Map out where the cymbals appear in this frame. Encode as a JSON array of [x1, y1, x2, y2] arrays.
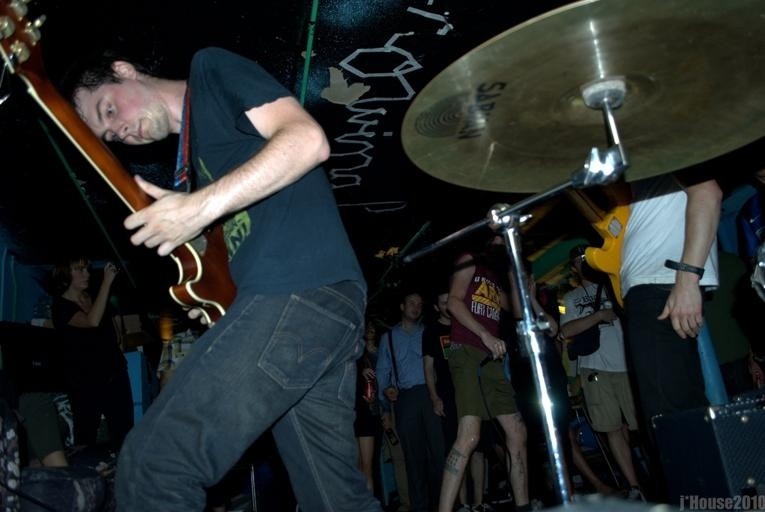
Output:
[[402, 0, 765, 195]]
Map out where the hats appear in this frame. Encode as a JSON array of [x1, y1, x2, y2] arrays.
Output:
[[570, 244, 589, 260]]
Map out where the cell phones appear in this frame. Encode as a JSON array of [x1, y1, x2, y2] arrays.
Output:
[[385, 428, 399, 446]]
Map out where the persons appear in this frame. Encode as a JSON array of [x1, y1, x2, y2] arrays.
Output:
[[52, 238, 133, 474], [62, 41, 387, 511], [156, 325, 201, 393], [17, 387, 79, 472]]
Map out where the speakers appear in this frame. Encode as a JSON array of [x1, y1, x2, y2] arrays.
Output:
[[0, 320, 70, 409], [650, 397, 765, 512]]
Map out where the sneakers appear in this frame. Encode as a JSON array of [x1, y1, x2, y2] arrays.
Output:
[[455, 502, 494, 511], [626, 486, 644, 502]]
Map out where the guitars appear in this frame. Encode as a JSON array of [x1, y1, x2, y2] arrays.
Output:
[[565, 187, 631, 307], [0, 0, 236, 328]]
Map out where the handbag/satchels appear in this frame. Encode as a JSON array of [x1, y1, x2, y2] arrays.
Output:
[[567, 321, 600, 361]]
